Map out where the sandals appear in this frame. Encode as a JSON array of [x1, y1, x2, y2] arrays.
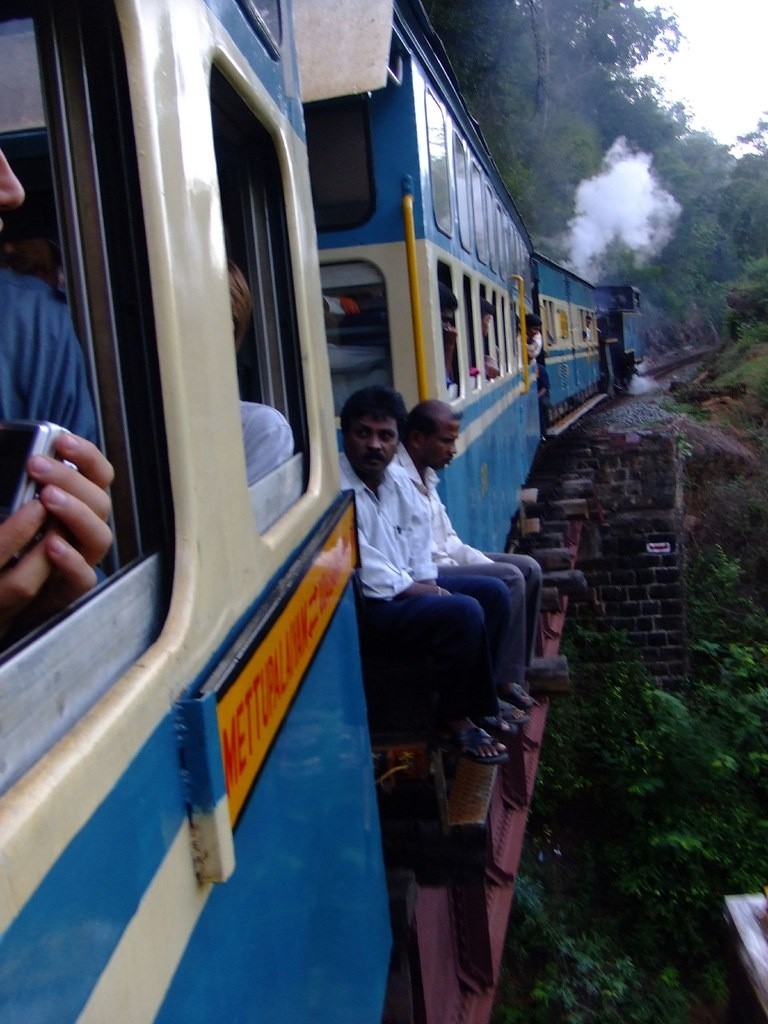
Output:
[[497, 698, 528, 724], [504, 683, 534, 709]]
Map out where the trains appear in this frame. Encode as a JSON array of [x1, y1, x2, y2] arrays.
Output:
[[1, 0, 644, 1024]]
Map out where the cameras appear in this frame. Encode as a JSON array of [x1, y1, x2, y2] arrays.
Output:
[[0, 419, 80, 562]]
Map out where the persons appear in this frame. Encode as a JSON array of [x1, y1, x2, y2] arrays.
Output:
[[0, 152, 593, 640], [395, 401, 542, 725], [336, 385, 522, 766]]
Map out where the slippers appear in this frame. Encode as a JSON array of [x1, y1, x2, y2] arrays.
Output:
[[471, 714, 516, 738], [430, 717, 510, 765]]
